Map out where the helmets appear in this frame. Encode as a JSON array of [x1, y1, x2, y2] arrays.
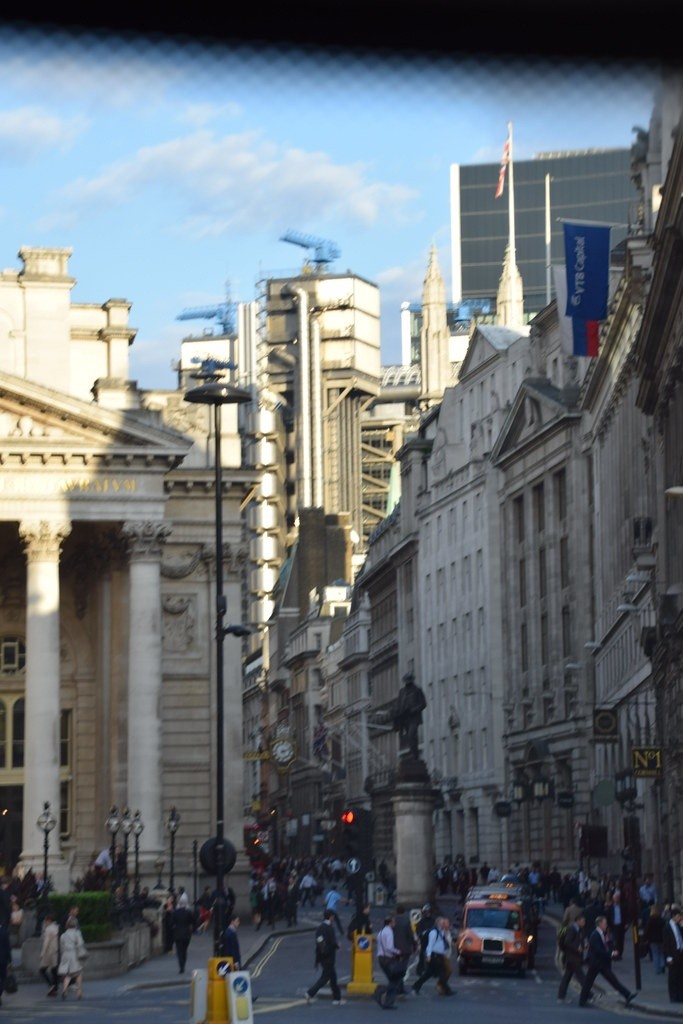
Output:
[[421, 903, 432, 915]]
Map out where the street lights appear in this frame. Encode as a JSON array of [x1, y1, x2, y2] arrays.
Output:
[[106, 805, 120, 897], [129, 810, 145, 896], [34, 801, 57, 935], [169, 805, 182, 898], [187, 379, 252, 884], [119, 809, 132, 899]]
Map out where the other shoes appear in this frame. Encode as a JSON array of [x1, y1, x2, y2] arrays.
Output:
[[588, 992, 603, 1002], [47, 983, 86, 1002], [332, 999, 348, 1007], [578, 1000, 596, 1009], [381, 1003, 397, 1010], [305, 992, 313, 1006], [625, 991, 640, 1004], [554, 996, 574, 1003], [410, 985, 423, 995], [442, 989, 458, 997], [178, 966, 185, 973], [373, 987, 382, 1006]]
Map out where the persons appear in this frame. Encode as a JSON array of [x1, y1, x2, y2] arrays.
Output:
[[37, 912, 58, 995], [57, 916, 86, 1000], [395, 903, 453, 994], [303, 909, 347, 1006], [58, 904, 80, 933], [434, 856, 682, 974], [555, 914, 599, 1003], [395, 672, 426, 756], [222, 914, 257, 1002], [0, 844, 149, 933], [578, 915, 638, 1007], [376, 915, 402, 1008], [163, 852, 397, 974], [37, 906, 50, 945], [663, 908, 682, 1003], [0, 919, 12, 1006]]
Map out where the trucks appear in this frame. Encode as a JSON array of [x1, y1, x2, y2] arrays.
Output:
[[458, 882, 544, 976]]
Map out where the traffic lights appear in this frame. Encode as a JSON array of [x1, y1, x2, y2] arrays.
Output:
[[337, 805, 371, 831]]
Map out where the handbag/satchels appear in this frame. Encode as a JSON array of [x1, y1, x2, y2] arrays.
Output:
[[385, 952, 402, 980], [74, 929, 90, 961], [5, 964, 19, 993]]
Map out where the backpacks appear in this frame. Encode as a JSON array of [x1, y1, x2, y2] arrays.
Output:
[[420, 926, 439, 951], [314, 924, 332, 958], [555, 926, 568, 951]]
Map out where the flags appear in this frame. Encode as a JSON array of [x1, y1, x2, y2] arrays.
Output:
[[562, 223, 609, 319], [495, 141, 508, 197], [572, 318, 598, 356], [313, 725, 328, 754]]
